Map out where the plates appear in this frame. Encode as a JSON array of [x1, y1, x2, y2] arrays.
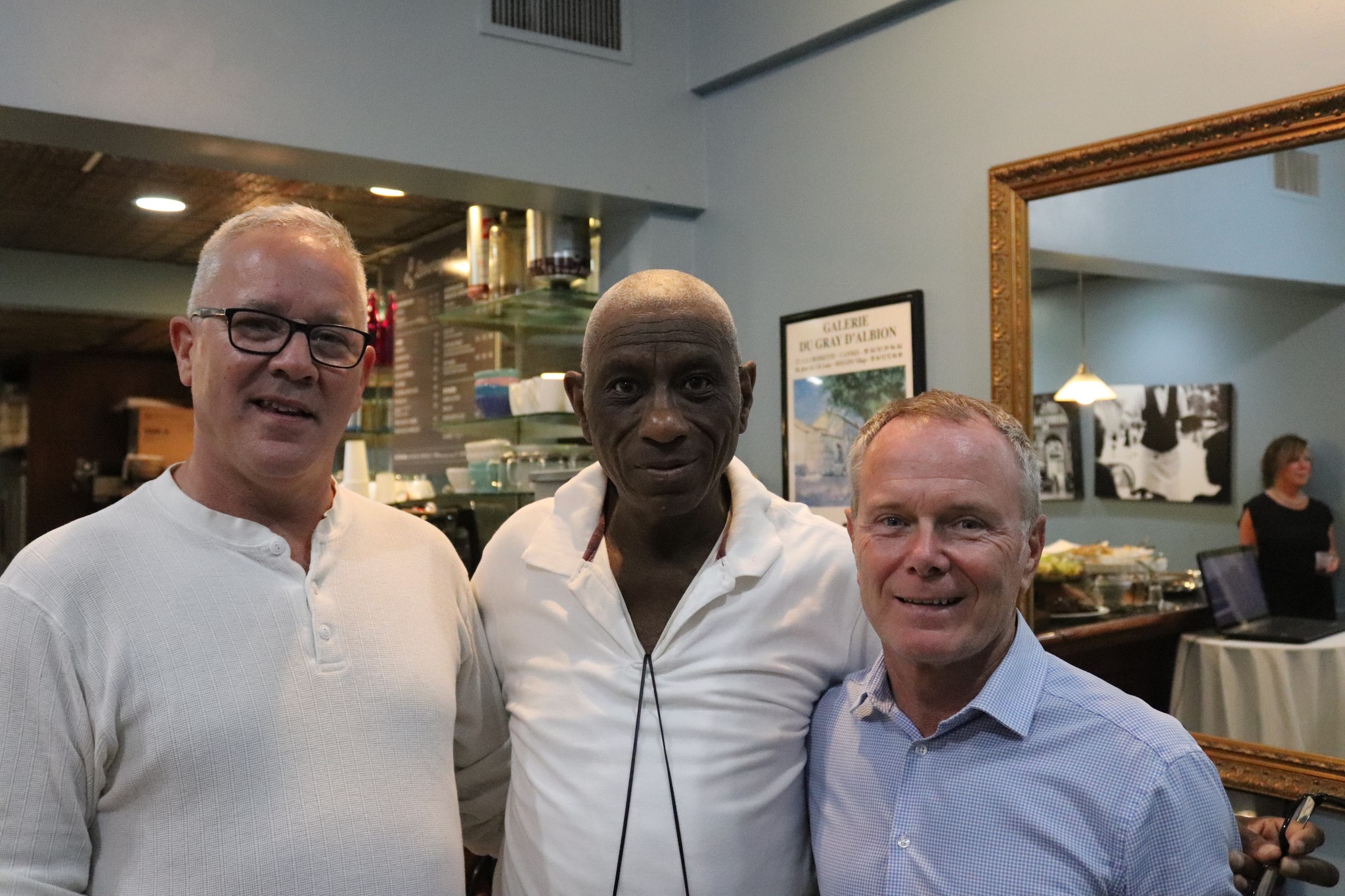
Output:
[[1157, 571, 1203, 595], [1050, 605, 1111, 618]]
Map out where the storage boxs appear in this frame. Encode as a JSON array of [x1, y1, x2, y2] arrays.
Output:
[[120, 407, 194, 462]]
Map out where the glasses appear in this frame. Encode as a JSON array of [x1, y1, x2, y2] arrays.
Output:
[[1249, 788, 1345, 896], [189, 305, 375, 369]]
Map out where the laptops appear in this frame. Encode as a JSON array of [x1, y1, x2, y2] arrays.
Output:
[[1196, 546, 1345, 645]]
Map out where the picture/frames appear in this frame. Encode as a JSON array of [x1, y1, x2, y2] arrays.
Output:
[[778, 289, 928, 525]]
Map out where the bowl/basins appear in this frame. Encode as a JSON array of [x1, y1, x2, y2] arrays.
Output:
[[473, 369, 518, 417], [445, 466, 473, 494]]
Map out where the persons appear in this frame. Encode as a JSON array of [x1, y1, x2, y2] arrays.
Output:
[[811, 388, 1243, 896], [467, 268, 1340, 896], [1236, 433, 1340, 621], [0, 204, 513, 896]]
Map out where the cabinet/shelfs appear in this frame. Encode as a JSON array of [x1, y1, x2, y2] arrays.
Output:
[[433, 287, 600, 439]]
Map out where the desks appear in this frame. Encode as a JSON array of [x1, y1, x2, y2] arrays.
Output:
[[1169, 634, 1345, 761], [1033, 607, 1216, 715]]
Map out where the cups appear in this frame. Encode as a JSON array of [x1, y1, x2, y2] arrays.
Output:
[[342, 439, 395, 505], [508, 378, 574, 415], [467, 456, 592, 493]]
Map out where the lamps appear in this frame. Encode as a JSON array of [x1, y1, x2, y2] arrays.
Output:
[[1053, 272, 1118, 407]]
